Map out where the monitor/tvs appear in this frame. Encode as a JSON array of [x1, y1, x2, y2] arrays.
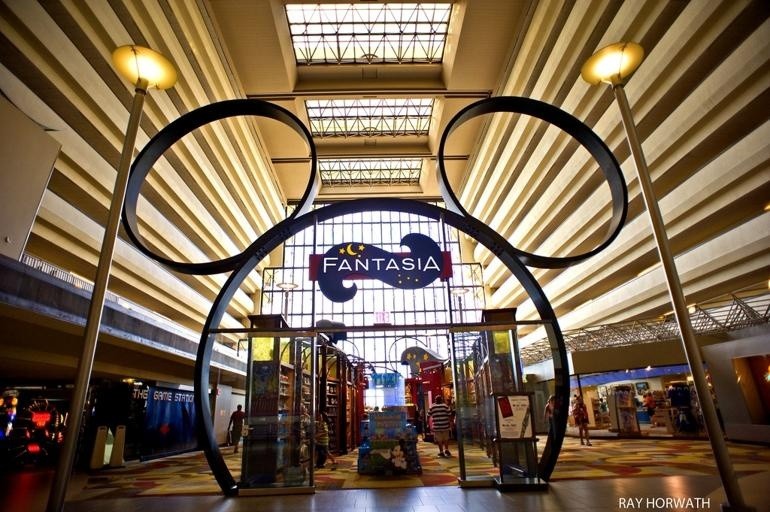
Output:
[[636, 383, 648, 390]]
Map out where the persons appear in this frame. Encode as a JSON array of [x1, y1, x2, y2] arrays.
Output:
[[598, 398, 608, 415], [311, 411, 338, 471], [543, 395, 556, 424], [227, 404, 246, 454], [642, 391, 658, 428], [572, 395, 593, 446], [425, 395, 453, 457]]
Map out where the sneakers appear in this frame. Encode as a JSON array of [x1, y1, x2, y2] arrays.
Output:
[[580, 441, 592, 446], [437, 450, 452, 457]]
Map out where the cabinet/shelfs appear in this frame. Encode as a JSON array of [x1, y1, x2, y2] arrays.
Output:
[[240, 359, 359, 485], [474, 353, 537, 476]]
